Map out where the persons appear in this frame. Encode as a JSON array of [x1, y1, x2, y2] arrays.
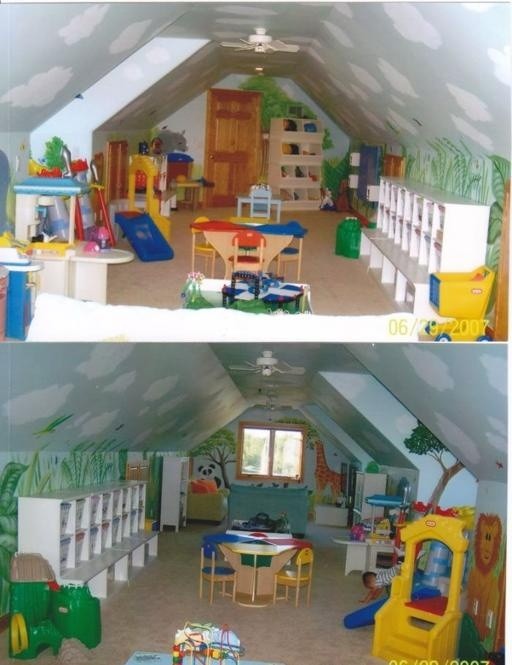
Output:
[[357, 564, 400, 604]]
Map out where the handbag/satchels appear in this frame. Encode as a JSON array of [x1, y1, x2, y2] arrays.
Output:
[[245, 513, 277, 532]]
